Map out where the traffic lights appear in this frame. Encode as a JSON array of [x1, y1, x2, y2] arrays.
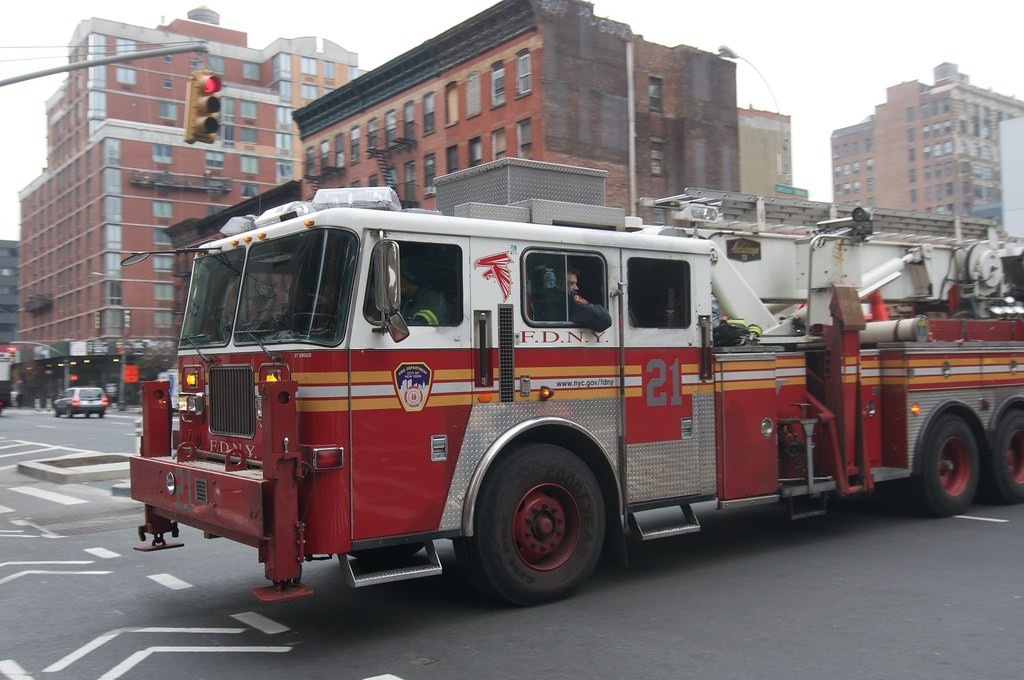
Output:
[[183, 70, 223, 144]]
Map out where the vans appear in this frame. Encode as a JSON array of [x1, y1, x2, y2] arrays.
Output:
[[53, 388, 108, 418]]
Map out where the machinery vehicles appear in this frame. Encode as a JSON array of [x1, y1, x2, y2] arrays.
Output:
[[128, 183, 1024, 605]]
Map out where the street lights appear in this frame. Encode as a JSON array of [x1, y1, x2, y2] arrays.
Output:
[[718, 45, 792, 190], [92, 272, 127, 411]]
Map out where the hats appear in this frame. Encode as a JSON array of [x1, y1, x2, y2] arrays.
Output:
[[404, 259, 432, 289]]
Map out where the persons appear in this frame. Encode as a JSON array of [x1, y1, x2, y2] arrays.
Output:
[[308, 255, 456, 325], [535, 266, 612, 332]]
